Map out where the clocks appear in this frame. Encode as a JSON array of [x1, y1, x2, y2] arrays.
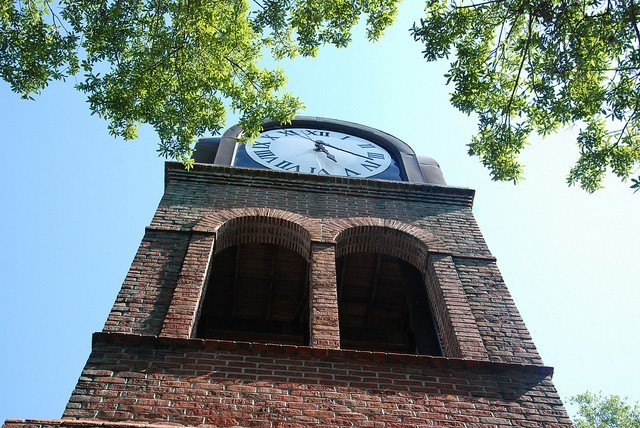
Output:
[[230, 120, 409, 182]]
